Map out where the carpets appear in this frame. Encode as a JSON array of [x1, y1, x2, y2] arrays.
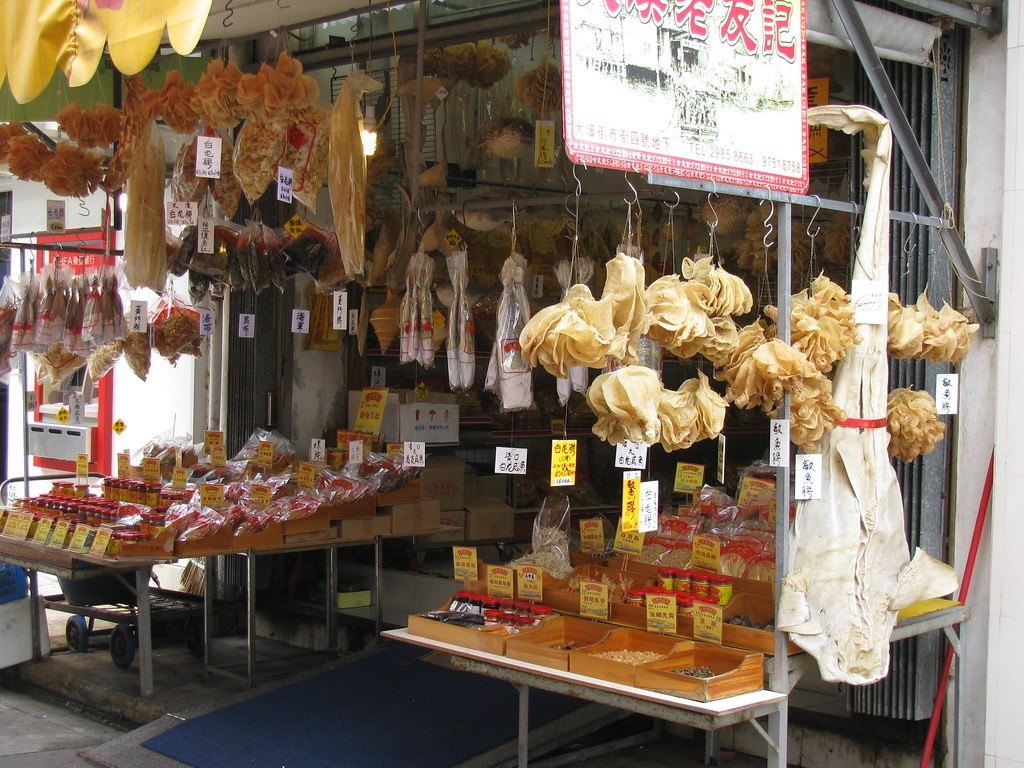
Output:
[[141, 637, 590, 768]]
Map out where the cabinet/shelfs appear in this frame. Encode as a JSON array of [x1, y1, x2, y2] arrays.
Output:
[[352, 278, 772, 521]]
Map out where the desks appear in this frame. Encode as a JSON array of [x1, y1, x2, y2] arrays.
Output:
[[0, 535, 385, 695], [447, 653, 811, 767]]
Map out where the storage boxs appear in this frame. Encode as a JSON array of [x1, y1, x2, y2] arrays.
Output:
[[209, 386, 527, 542]]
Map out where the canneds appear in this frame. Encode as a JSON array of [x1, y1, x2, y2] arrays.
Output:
[[452, 591, 551, 634], [624, 567, 733, 617], [0, 476, 187, 555]]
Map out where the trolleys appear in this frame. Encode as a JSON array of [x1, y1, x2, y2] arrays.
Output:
[[0, 472, 238, 667]]
